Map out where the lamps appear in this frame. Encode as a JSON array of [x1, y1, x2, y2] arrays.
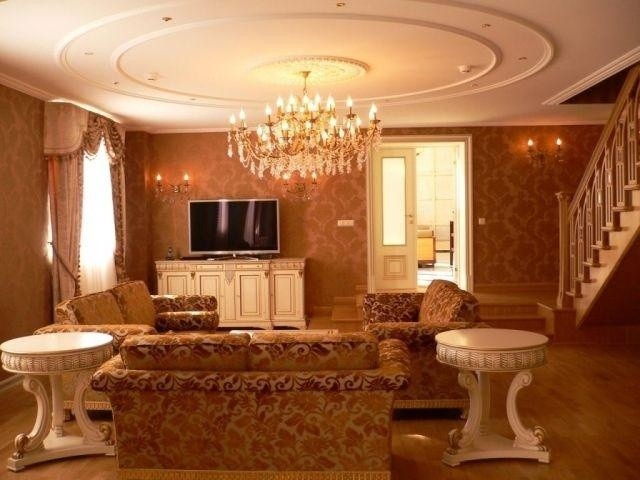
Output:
[[228, 53, 382, 176], [281, 172, 321, 205], [154, 172, 193, 206]]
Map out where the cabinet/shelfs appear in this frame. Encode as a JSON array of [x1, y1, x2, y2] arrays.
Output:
[[225, 259, 273, 331], [155, 258, 224, 330], [267, 258, 309, 331]]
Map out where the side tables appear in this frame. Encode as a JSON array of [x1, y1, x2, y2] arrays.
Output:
[[0, 331, 115, 472], [435, 328, 551, 467]]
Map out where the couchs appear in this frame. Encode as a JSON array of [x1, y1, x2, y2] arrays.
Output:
[[363, 280, 494, 420], [34, 280, 219, 421], [90, 331, 411, 479], [526, 137, 565, 177]]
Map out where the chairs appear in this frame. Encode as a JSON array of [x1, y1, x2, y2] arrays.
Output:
[[415, 231, 436, 267]]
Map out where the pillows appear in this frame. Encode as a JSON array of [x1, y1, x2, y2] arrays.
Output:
[[156, 311, 218, 330]]
